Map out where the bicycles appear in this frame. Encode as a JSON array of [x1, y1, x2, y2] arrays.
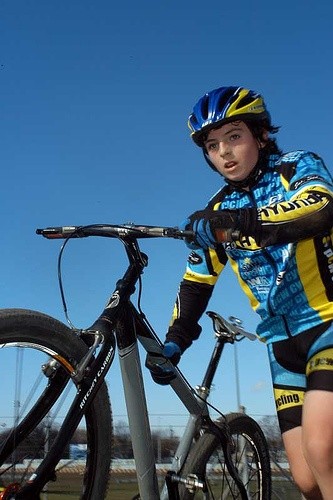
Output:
[[0, 222, 273, 500]]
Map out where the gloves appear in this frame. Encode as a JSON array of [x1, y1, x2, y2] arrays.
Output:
[[145, 331, 192, 386], [184, 207, 258, 250]]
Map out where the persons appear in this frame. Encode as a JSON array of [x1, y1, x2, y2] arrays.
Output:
[[145, 86, 333, 500]]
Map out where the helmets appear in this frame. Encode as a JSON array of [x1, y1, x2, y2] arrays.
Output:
[[188, 86, 266, 147]]
